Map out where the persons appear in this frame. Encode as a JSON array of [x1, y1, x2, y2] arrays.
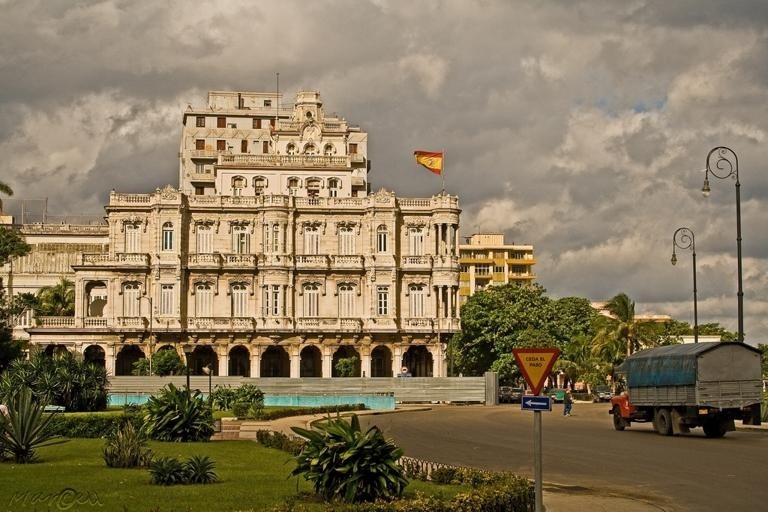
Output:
[[562, 388, 572, 417]]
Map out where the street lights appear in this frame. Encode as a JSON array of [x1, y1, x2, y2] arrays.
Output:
[[201, 363, 213, 422], [701, 145, 744, 345], [670, 227, 698, 344]]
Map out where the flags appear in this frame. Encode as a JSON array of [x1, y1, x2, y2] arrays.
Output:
[[413, 149, 443, 176]]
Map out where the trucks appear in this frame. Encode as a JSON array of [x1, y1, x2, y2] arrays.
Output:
[[607, 342, 763, 438]]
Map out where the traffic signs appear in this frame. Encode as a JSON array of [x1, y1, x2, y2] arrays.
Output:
[[511, 346, 560, 395], [520, 396, 550, 412]]
